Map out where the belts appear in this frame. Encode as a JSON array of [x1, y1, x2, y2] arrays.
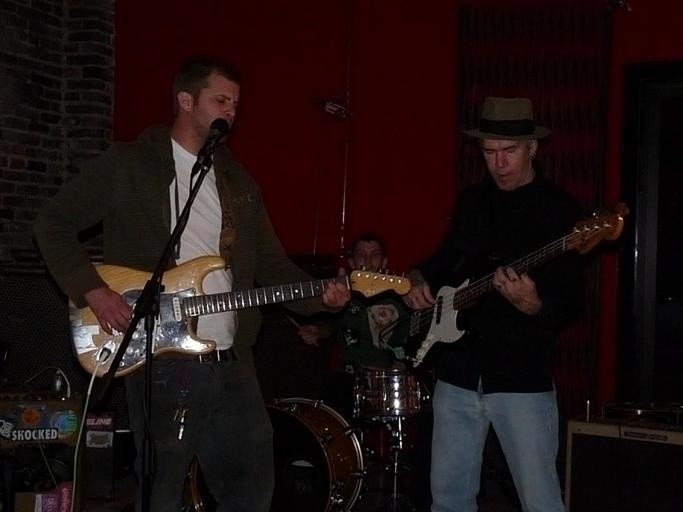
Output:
[[197, 346, 237, 364]]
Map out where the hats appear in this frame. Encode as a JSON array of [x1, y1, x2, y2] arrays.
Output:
[[457, 95, 554, 141]]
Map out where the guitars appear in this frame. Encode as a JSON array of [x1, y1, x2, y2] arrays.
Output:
[[378, 203, 630, 366], [70, 256, 411, 378]]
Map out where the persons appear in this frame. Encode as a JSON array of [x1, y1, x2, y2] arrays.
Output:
[[296, 231, 413, 428], [397, 97, 598, 512], [31, 53, 353, 512]]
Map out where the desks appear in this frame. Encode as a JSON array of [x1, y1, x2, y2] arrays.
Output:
[[564, 419, 682, 512]]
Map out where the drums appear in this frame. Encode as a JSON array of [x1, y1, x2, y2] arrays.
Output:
[[356, 368, 424, 419], [188, 397, 366, 509]]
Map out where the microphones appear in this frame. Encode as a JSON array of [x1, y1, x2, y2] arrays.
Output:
[[191, 118, 229, 176]]
